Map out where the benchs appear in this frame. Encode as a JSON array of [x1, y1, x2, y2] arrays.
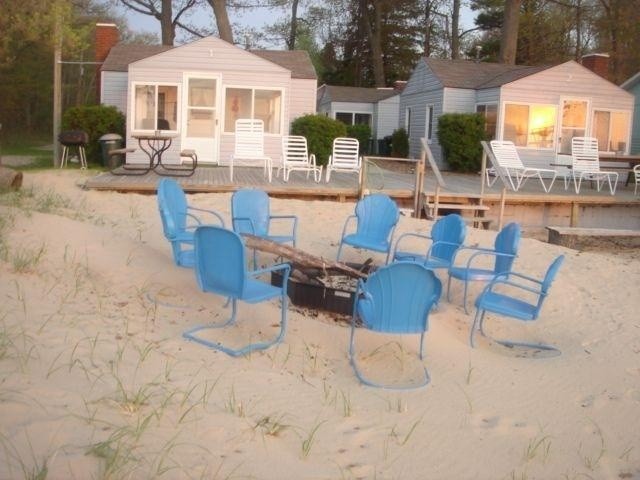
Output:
[[550, 163, 640, 174], [106, 147, 139, 176], [180, 148, 198, 178]]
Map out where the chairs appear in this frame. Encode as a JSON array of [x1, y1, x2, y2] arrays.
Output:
[[182, 225, 290, 358], [349, 262, 442, 390], [471, 256, 565, 359], [633, 164, 640, 194], [481, 139, 558, 192], [278, 136, 324, 183], [564, 137, 619, 196], [322, 136, 363, 182], [335, 192, 520, 315], [228, 118, 273, 182], [157, 178, 298, 269]]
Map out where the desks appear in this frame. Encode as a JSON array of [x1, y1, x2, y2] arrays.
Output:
[[131, 131, 180, 173], [558, 151, 640, 189]]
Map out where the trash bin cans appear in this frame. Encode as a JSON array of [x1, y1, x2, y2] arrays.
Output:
[[368, 136, 393, 157], [99, 133, 123, 168]]
